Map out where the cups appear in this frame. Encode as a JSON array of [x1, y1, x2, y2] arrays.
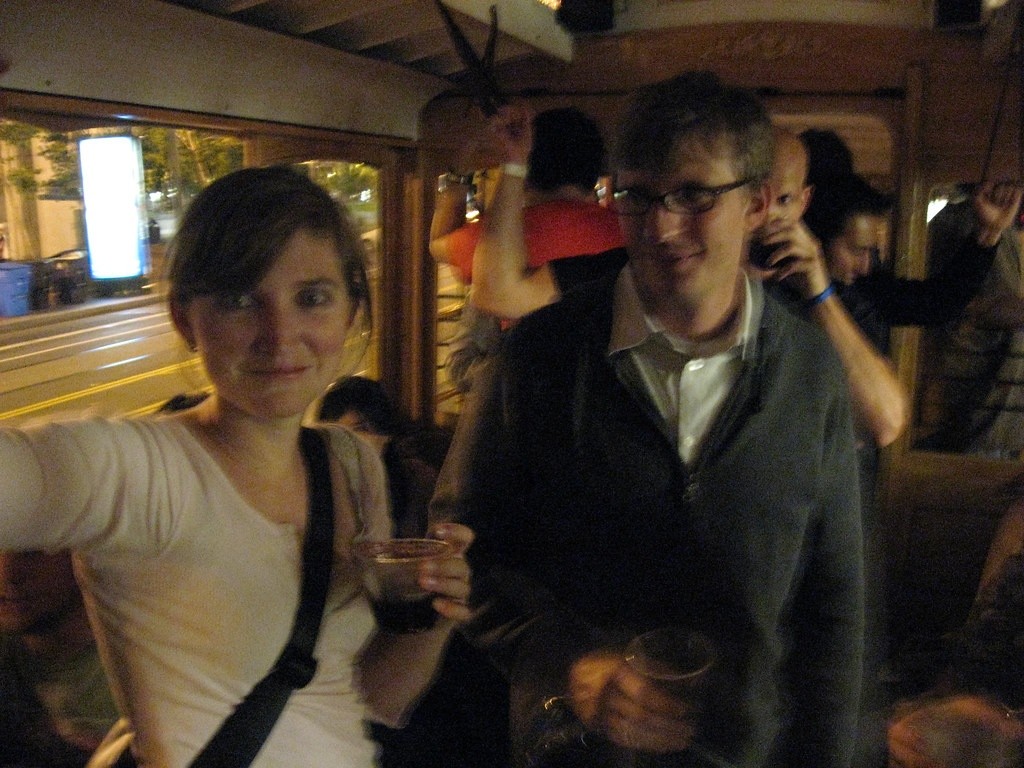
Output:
[[622, 627, 719, 714], [350, 538, 454, 636], [748, 232, 797, 271], [890, 692, 1022, 768]]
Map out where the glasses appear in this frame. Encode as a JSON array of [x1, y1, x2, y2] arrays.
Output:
[[607, 177, 758, 218]]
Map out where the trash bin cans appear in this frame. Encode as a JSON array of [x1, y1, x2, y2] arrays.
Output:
[[0, 262, 33, 317]]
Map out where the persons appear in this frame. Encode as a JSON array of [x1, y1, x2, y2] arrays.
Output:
[[0, 71, 1023, 768]]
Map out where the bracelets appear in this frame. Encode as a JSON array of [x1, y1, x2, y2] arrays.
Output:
[[439, 171, 471, 186], [801, 276, 838, 307], [502, 162, 529, 177]]
